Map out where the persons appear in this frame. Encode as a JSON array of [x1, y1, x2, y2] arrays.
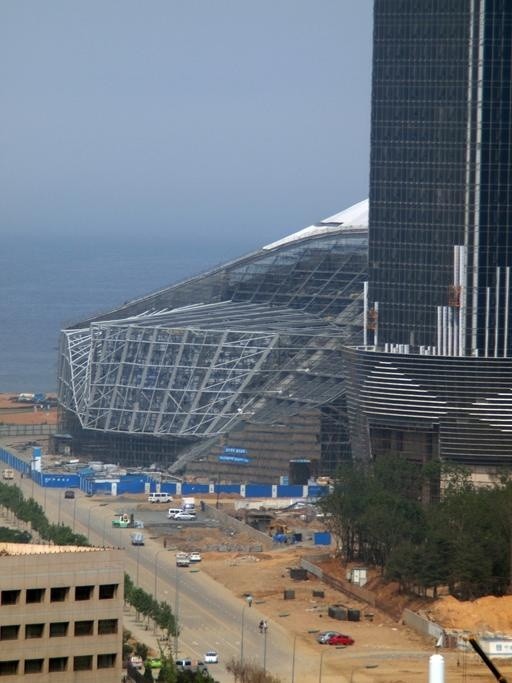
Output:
[[258, 620, 263, 633], [247, 594, 252, 608], [263, 620, 268, 633]]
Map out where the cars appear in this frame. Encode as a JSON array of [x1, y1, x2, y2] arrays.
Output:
[[316, 630, 340, 644], [144, 655, 164, 669], [190, 551, 202, 561], [39, 396, 58, 407], [174, 511, 197, 520], [202, 650, 221, 664], [329, 633, 356, 646]]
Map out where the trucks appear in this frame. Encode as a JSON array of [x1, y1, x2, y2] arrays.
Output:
[[174, 551, 192, 567], [128, 532, 145, 544], [181, 497, 196, 514]]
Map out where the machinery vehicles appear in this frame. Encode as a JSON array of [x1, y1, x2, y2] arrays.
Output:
[[460, 631, 509, 682], [16, 390, 46, 403], [268, 500, 327, 544], [112, 511, 136, 528]]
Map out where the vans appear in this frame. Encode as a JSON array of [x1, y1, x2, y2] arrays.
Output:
[[168, 507, 184, 519], [126, 653, 143, 670], [148, 492, 174, 503], [175, 658, 210, 676], [2, 468, 14, 479]]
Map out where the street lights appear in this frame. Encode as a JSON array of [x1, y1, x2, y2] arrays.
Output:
[[101, 511, 124, 547], [240, 600, 266, 669], [262, 611, 290, 676], [135, 535, 160, 617], [292, 626, 321, 682], [173, 567, 200, 660], [318, 644, 348, 682], [350, 663, 378, 682], [18, 457, 110, 545], [117, 522, 140, 551], [152, 545, 177, 631]]
